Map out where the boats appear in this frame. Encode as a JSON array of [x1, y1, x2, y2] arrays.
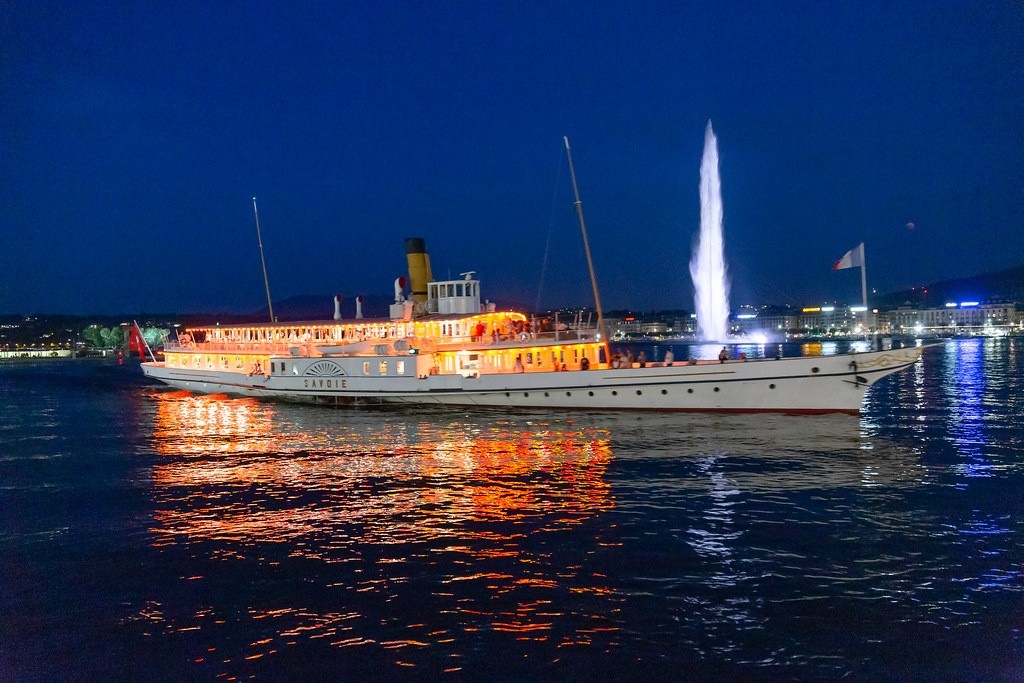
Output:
[[140, 136, 922, 412]]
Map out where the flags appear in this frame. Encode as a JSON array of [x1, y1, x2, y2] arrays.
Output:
[[128, 324, 147, 363], [167, 330, 177, 342], [834, 244, 861, 270]]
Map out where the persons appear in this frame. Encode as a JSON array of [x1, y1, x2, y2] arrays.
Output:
[[610, 348, 634, 369], [553, 358, 559, 372], [600, 348, 605, 363], [738, 353, 746, 359], [253, 361, 264, 374], [561, 365, 567, 371], [470, 323, 476, 342], [664, 349, 674, 367], [719, 347, 728, 363], [581, 354, 589, 371], [638, 352, 646, 368], [513, 357, 524, 373], [492, 318, 549, 342], [476, 320, 484, 342]]
